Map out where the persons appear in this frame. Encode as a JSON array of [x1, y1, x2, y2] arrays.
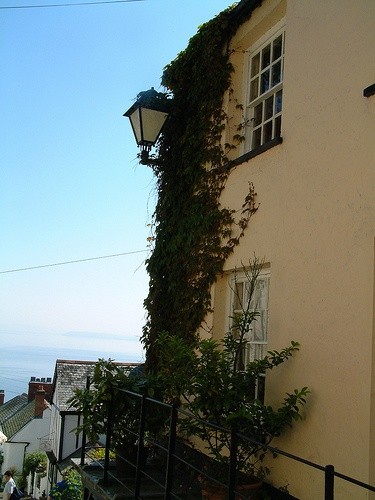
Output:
[[2, 470, 22, 500]]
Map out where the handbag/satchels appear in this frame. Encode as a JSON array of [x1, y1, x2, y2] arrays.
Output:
[[8, 487, 25, 500]]
